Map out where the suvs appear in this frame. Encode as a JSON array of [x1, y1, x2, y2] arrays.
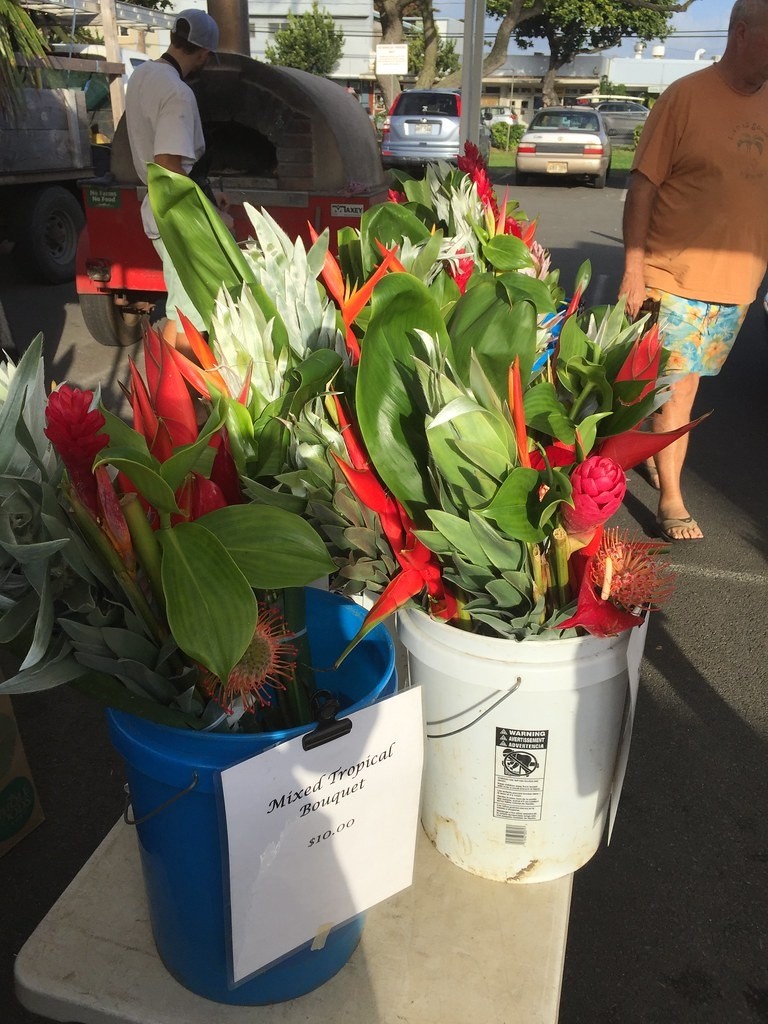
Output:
[[379, 88, 493, 172], [584, 101, 651, 137]]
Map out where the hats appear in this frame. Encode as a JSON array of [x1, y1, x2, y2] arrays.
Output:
[[171, 8, 222, 67]]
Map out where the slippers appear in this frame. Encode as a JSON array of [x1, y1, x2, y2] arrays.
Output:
[[655, 513, 705, 544], [642, 463, 660, 491]]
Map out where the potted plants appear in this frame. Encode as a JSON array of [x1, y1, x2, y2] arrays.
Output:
[[1, 157, 404, 1005], [296, 137, 716, 884]]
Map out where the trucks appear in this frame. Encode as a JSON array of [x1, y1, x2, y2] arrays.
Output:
[[0, 41, 151, 285]]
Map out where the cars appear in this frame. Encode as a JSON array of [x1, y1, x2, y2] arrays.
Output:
[[515, 105, 619, 190], [480, 105, 518, 127]]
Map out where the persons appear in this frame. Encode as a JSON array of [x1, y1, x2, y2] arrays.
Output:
[[125, 9, 234, 432], [614, 1, 768, 544]]
